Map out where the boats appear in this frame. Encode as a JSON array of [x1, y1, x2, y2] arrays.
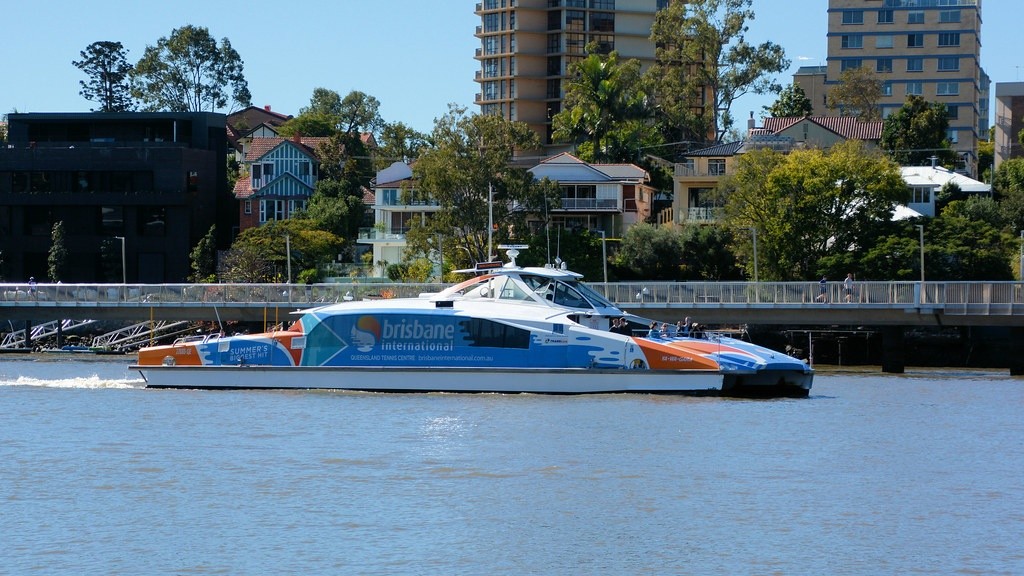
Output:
[[127, 187, 815, 398]]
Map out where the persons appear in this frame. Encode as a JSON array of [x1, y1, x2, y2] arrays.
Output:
[[618, 317, 632, 336], [218, 328, 228, 339], [608, 318, 620, 334], [28, 276, 39, 301], [659, 323, 670, 337], [676, 317, 709, 338], [816, 275, 828, 304], [648, 321, 667, 338], [843, 273, 855, 304]]
